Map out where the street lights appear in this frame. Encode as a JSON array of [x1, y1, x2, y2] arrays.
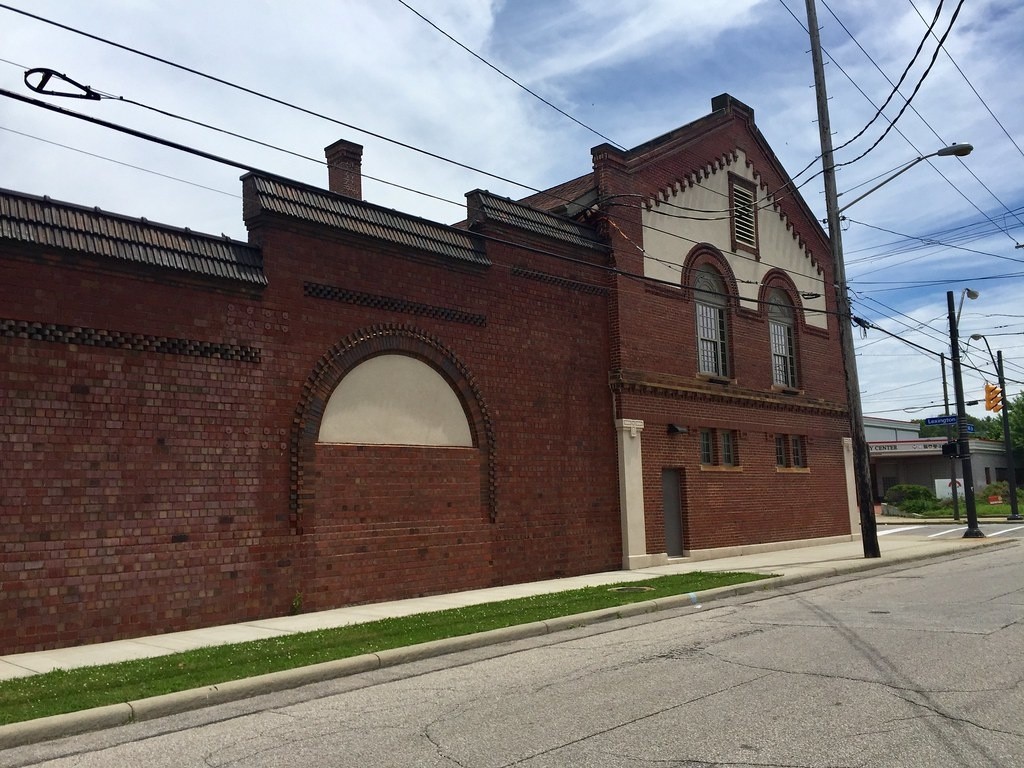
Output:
[[971, 333, 1024, 520], [818, 142, 974, 559], [943, 287, 987, 538]]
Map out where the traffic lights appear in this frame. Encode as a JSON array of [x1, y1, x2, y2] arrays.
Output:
[[985, 383, 997, 411], [992, 388, 1004, 413]]
